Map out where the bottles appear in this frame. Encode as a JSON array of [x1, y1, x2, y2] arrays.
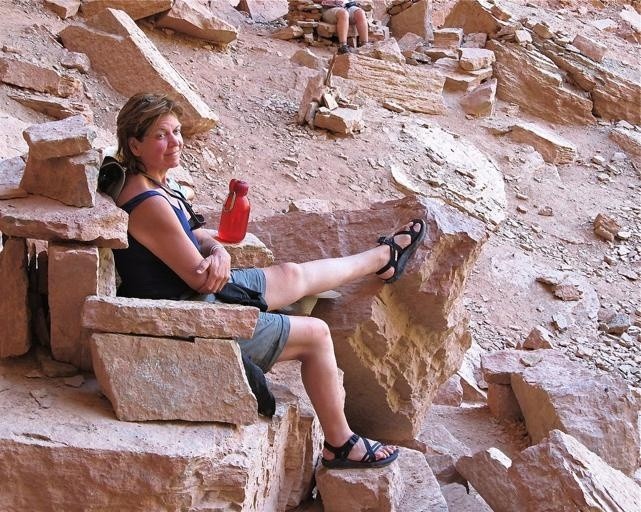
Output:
[[217, 178, 252, 243]]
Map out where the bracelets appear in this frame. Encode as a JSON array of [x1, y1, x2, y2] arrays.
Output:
[[209, 244, 224, 255]]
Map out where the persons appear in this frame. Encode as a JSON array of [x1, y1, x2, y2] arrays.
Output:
[[313, 1, 375, 56], [112, 90, 428, 472]]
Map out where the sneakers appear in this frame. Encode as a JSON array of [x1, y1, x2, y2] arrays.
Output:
[[337, 45, 350, 54]]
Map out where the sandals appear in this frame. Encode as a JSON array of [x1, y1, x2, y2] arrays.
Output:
[[321, 432, 400, 469], [375, 217, 427, 284]]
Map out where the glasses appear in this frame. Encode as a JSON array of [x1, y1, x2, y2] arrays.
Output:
[[172, 190, 206, 230]]
